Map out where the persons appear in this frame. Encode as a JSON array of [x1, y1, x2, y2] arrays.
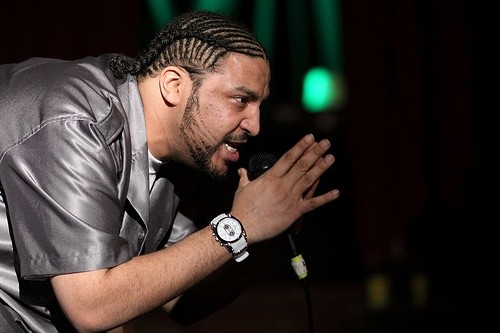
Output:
[[0, 10, 339, 332]]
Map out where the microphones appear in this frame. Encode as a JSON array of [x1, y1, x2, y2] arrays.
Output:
[[249, 154, 311, 285]]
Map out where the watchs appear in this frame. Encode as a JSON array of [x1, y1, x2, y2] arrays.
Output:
[[209, 213, 250, 262]]
